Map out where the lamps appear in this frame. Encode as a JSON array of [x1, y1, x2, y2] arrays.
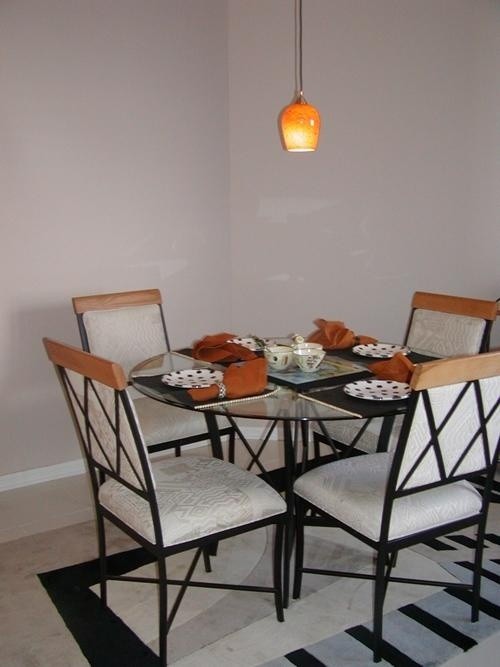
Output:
[[279, 0, 320, 153]]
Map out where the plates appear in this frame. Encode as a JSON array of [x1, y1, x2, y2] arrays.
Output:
[[343, 379, 411, 401], [352, 344, 411, 359], [226, 338, 277, 352], [161, 369, 224, 388]]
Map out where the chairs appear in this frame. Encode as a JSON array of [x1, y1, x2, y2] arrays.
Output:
[[293, 352, 500, 663], [42, 335, 287, 664], [312, 290, 498, 461], [71, 289, 237, 486]]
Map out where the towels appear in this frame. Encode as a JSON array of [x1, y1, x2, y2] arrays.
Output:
[[188, 357, 268, 403], [367, 352, 417, 383], [305, 319, 377, 350], [191, 333, 257, 363]]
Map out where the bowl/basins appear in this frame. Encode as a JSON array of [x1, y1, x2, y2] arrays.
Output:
[[293, 350, 326, 373], [264, 346, 293, 370], [296, 342, 323, 350]]
